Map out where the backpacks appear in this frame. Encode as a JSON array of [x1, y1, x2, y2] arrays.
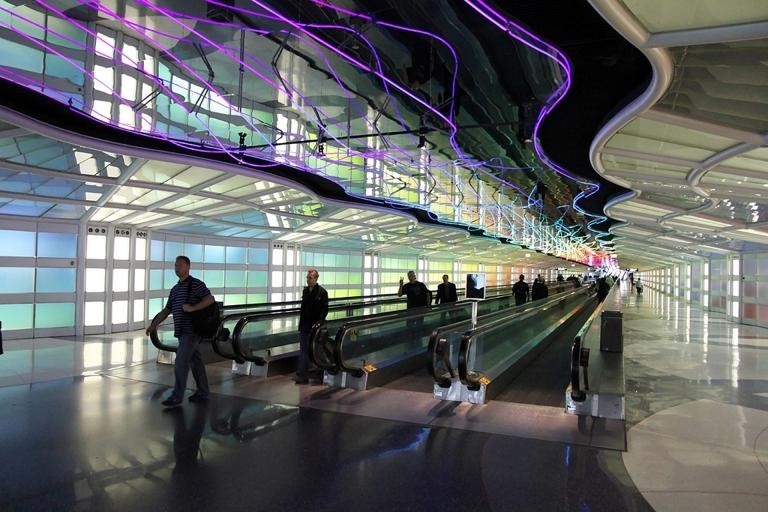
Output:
[[185, 277, 222, 339]]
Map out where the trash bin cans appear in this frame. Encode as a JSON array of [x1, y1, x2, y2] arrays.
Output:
[[640, 285, 643, 292], [600, 311, 624, 353]]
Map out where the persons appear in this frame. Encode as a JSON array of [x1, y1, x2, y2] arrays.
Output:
[[635, 278, 641, 297], [434, 274, 457, 326], [160, 406, 209, 512], [396, 270, 430, 356], [531, 273, 617, 303], [313, 2, 467, 155], [511, 274, 529, 312], [145, 254, 216, 406], [288, 268, 328, 387]]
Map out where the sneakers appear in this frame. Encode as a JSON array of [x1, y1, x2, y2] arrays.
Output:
[[188, 390, 210, 403], [291, 374, 310, 383], [161, 393, 183, 407]]
